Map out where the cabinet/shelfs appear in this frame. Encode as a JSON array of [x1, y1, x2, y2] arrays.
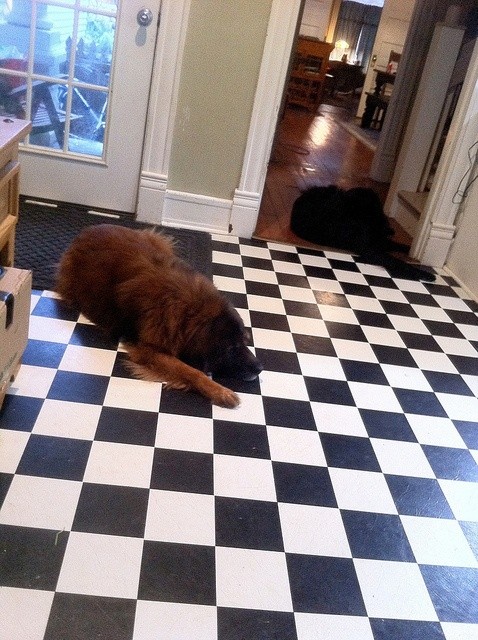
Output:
[[285, 40, 334, 114]]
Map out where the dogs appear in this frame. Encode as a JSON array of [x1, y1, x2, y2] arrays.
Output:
[[288, 182, 438, 283], [53, 222, 265, 410]]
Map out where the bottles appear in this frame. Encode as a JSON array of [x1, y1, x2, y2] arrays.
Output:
[[370, 53, 377, 68]]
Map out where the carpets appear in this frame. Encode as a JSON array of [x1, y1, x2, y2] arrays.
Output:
[[1, 201, 212, 292]]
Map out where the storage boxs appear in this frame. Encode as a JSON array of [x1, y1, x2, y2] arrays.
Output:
[[0, 266, 32, 389]]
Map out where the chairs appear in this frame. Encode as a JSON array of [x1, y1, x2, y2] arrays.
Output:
[[372, 50, 402, 130], [0, 72, 84, 151]]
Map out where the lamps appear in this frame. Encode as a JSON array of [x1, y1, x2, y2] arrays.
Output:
[[335, 40, 349, 61]]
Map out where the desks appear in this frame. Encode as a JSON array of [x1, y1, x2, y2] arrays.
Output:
[[0, 115, 32, 266], [360, 69, 397, 130]]
